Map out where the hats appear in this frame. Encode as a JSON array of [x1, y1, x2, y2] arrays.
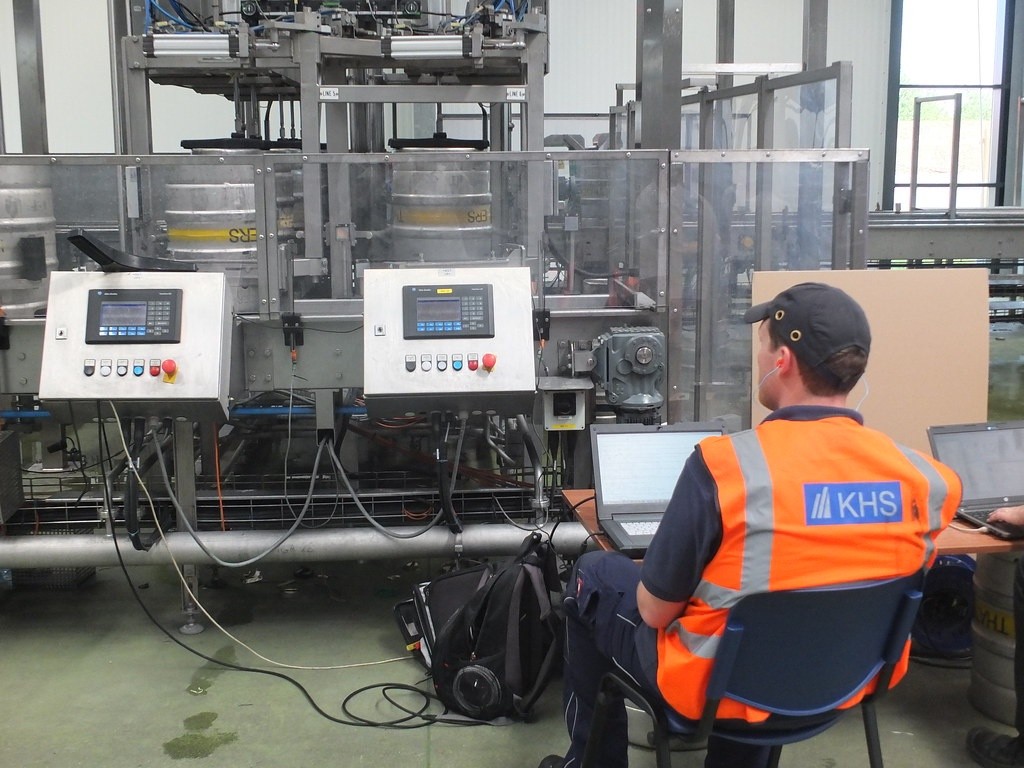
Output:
[[740, 282, 872, 376]]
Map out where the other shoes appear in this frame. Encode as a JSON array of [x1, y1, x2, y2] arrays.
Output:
[[966, 722, 1023, 767]]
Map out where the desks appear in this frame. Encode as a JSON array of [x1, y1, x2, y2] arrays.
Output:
[[559, 490, 1024, 565]]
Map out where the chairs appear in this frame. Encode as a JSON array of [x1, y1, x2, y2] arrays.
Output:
[[576, 546, 924, 768]]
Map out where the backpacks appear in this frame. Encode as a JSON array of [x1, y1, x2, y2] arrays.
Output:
[[429, 532, 559, 724]]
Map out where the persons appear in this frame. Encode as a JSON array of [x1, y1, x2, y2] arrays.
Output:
[[539, 282, 964, 768]]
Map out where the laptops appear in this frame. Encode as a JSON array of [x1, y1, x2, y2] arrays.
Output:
[[926, 420, 1024, 541], [589, 421, 728, 558]]
